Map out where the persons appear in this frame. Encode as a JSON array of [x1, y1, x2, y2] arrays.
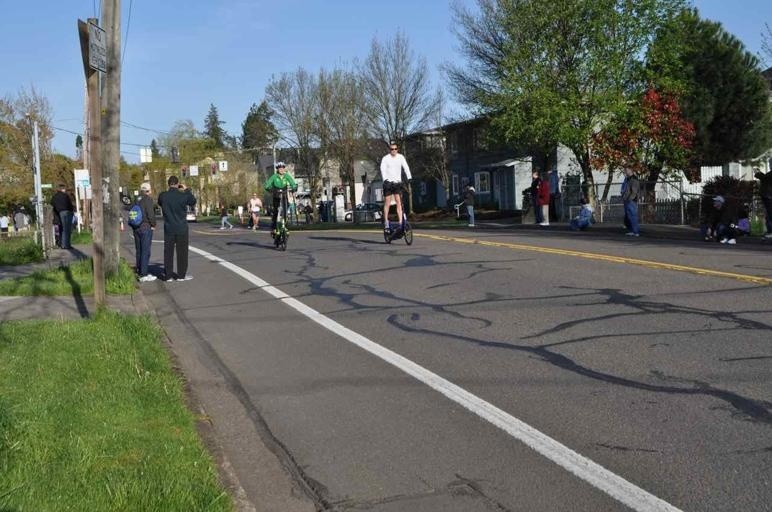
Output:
[[129, 183, 157, 281], [295, 202, 323, 223], [379, 143, 412, 234], [754, 159, 772, 238], [571, 198, 592, 230], [263, 162, 297, 236], [620, 166, 641, 236], [219, 192, 263, 232], [529, 167, 558, 227], [730, 209, 750, 236], [464, 183, 476, 226], [704, 194, 740, 244], [1, 184, 73, 250], [158, 176, 197, 282]]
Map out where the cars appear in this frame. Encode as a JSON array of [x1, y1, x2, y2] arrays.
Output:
[[344, 203, 380, 222], [187, 211, 196, 222]]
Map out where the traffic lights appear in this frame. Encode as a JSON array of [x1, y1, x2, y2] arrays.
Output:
[[180, 165, 187, 178], [211, 162, 217, 176]]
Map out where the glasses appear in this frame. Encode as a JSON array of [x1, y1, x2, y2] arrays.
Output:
[[390, 147, 398, 150]]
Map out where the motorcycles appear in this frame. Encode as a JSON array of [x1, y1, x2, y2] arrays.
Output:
[[266, 187, 294, 250], [382, 180, 414, 244]]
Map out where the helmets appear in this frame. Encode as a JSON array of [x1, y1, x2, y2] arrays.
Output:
[[276, 162, 285, 168]]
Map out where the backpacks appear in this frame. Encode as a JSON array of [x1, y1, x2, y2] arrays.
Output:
[[128, 196, 146, 227]]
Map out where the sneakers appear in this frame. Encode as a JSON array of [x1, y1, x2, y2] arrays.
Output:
[[139, 274, 157, 282], [177, 275, 193, 281], [167, 277, 174, 282], [705, 234, 736, 244]]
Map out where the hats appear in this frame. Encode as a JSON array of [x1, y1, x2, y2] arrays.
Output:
[[712, 196, 724, 203], [141, 183, 151, 191]]
